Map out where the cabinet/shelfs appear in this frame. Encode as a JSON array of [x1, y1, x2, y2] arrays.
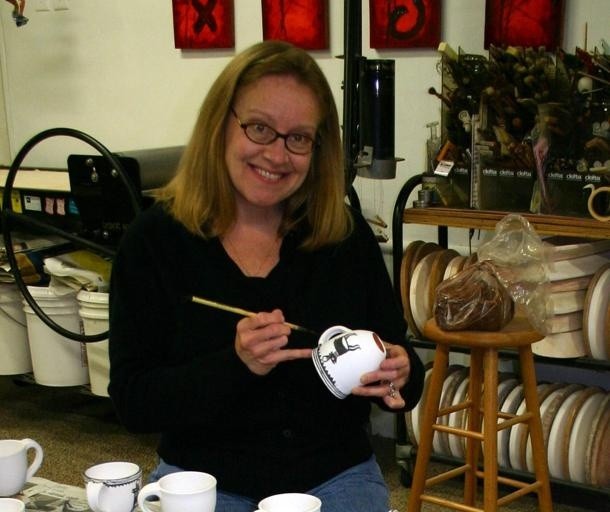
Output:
[[385, 165, 609, 510]]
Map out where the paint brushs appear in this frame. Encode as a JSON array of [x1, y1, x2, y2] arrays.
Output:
[[191, 295, 308, 332]]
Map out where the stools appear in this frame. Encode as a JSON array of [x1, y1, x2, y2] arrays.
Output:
[[407, 314, 553, 510]]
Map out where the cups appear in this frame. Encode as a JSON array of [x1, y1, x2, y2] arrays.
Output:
[[311, 323, 386, 402], [81, 461, 142, 512], [254, 492, 322, 512], [137, 469, 218, 511], [0, 498, 28, 511], [0, 437, 44, 495]]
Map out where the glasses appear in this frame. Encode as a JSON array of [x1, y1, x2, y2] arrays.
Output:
[[230, 106, 319, 155]]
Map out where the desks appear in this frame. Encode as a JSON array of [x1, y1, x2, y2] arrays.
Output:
[[1, 472, 158, 511]]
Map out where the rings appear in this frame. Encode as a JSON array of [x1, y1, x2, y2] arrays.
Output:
[[380, 380, 395, 397]]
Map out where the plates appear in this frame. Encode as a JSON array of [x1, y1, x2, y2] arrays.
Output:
[[398, 239, 610, 363], [406, 368, 610, 490]]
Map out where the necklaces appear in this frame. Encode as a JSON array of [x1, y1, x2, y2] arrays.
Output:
[[223, 229, 282, 276]]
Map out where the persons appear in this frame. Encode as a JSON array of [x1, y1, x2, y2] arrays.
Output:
[[108, 42, 425, 512]]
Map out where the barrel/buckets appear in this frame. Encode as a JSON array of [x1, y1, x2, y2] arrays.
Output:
[[21, 285, 91, 389], [1, 285, 32, 377], [78, 290, 110, 400]]
